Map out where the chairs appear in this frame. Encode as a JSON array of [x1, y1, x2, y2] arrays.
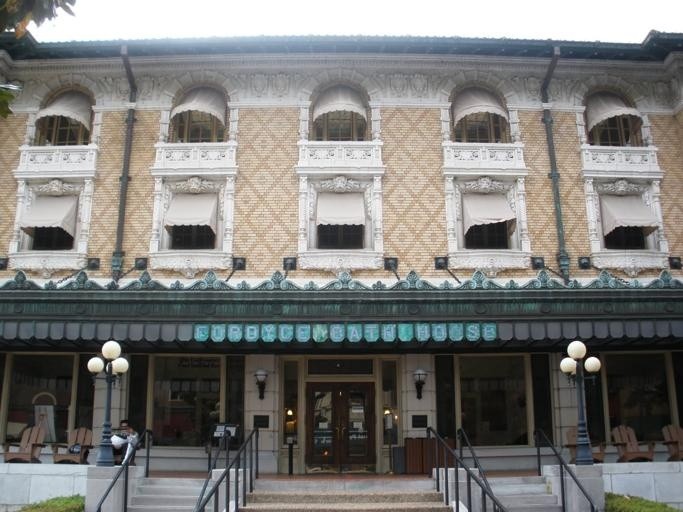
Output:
[[661, 424, 683, 461], [563, 428, 606, 463], [50, 423, 95, 465], [611, 425, 656, 462], [0, 423, 48, 464]]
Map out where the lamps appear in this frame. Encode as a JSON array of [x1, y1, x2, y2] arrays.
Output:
[[254, 369, 268, 400], [413, 368, 429, 400]]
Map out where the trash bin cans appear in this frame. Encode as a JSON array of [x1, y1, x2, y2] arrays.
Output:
[[279, 444, 301, 474], [379, 444, 398, 475]]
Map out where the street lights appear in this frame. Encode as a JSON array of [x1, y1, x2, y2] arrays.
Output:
[[559, 340, 602, 465], [87, 340, 129, 467]]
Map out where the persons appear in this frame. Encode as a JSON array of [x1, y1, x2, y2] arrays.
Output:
[[110, 420, 142, 466]]
